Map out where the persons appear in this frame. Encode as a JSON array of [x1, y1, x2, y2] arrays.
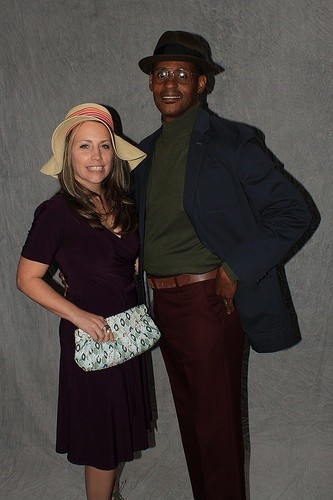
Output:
[[16, 103, 159, 500], [58, 31, 322, 500]]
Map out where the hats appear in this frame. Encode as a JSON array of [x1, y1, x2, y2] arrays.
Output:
[[40, 103, 148, 179], [138, 31, 225, 76]]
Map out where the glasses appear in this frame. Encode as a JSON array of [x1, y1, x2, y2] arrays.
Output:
[[151, 67, 201, 84]]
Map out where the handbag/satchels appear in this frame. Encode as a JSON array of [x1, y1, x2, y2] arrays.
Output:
[[75, 304, 161, 372]]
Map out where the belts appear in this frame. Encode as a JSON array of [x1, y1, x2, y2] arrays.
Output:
[[147, 269, 218, 289]]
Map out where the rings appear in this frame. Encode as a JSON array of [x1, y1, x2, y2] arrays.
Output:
[[102, 325, 111, 333]]
